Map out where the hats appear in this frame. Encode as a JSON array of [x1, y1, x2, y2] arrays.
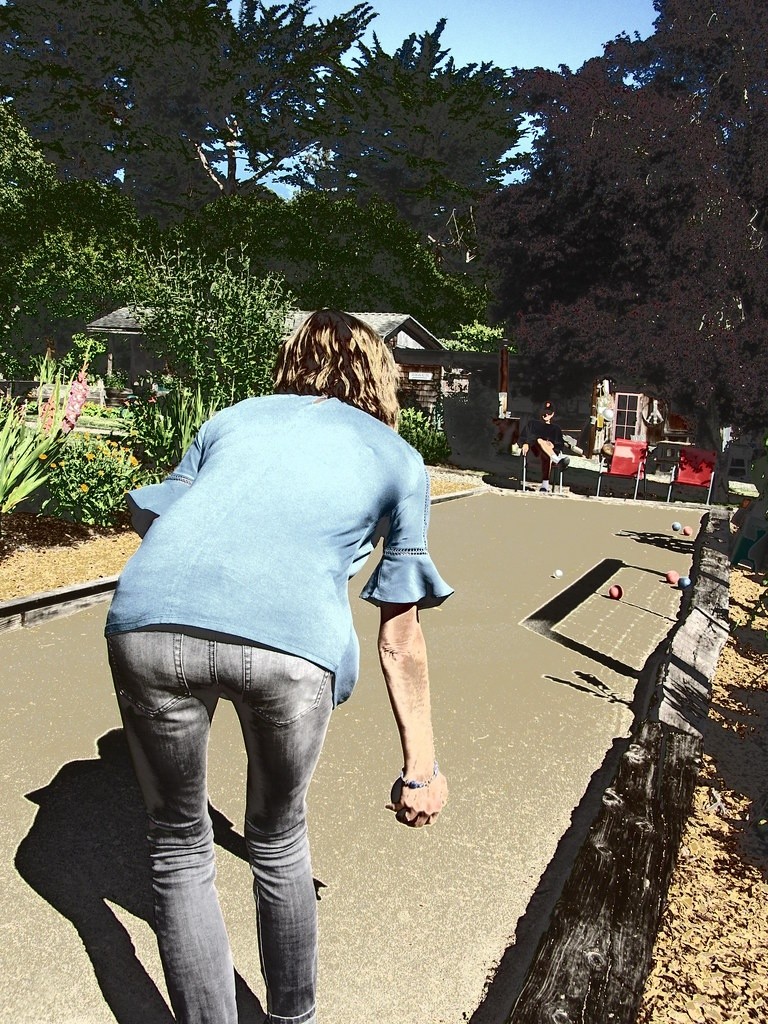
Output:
[[540, 400, 557, 414]]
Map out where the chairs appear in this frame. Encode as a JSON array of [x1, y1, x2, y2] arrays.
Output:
[[667, 447, 718, 505], [518, 447, 563, 493], [597, 438, 651, 501]]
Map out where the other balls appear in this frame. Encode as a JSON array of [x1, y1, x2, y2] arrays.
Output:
[[684, 526, 692, 535], [666, 570, 679, 584], [678, 577, 691, 589], [672, 522, 681, 531], [609, 584, 624, 599], [390, 777, 405, 803], [555, 569, 563, 577]]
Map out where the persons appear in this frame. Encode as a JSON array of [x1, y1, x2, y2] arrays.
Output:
[[103, 304, 455, 1024], [509, 400, 570, 493]]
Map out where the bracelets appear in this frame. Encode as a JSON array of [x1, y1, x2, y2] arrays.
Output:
[[399, 760, 439, 789]]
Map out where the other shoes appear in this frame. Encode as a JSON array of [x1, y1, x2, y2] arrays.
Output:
[[539, 480, 551, 493], [556, 456, 571, 473]]
